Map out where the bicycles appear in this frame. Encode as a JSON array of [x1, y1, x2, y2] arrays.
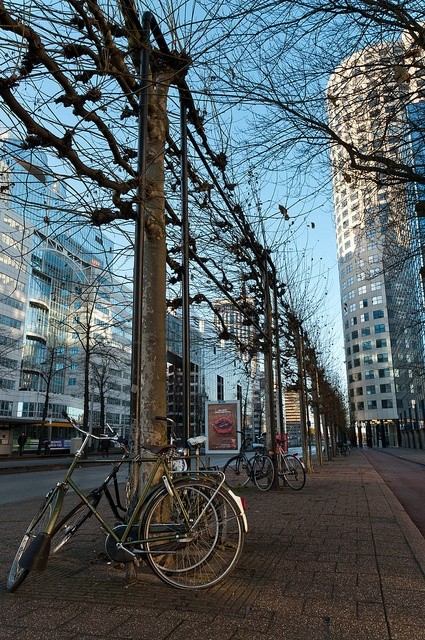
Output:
[[248, 432, 306, 491], [7, 410, 248, 591], [223, 430, 275, 491], [136, 414, 226, 578], [49, 421, 132, 575]]
